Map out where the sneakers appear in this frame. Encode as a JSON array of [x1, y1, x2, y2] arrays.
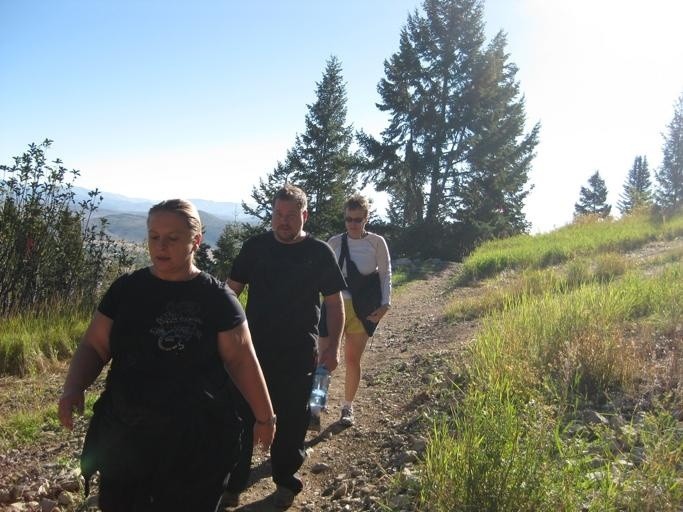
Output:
[[340, 406, 354, 425], [273, 476, 303, 495]]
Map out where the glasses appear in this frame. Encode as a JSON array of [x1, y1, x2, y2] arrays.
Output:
[[344, 216, 365, 224]]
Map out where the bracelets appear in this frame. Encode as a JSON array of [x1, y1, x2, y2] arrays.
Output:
[[385, 304, 392, 309]]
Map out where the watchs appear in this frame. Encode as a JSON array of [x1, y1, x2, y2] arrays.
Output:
[[255, 414, 277, 426]]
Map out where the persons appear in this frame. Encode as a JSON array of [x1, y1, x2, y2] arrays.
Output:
[[58, 198, 277, 512], [319, 198, 392, 426], [227, 187, 346, 507]]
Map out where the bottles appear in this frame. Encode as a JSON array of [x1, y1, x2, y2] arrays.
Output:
[[308, 363, 329, 408]]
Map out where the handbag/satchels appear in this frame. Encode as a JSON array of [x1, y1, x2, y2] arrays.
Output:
[[345, 261, 382, 319], [189, 364, 256, 446]]
